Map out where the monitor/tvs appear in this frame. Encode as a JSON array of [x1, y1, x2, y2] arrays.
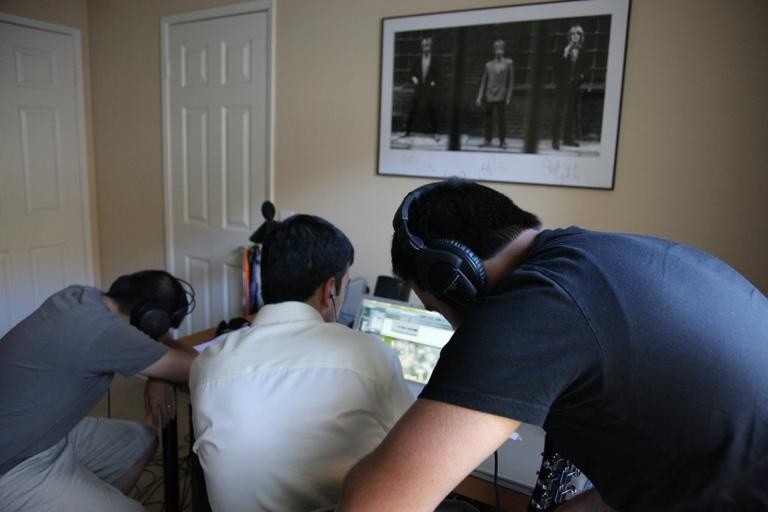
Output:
[[350, 293, 456, 397]]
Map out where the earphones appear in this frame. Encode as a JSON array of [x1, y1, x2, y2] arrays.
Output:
[[328, 291, 335, 302]]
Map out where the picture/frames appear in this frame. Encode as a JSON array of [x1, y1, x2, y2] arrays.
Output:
[[373, 0, 632, 192]]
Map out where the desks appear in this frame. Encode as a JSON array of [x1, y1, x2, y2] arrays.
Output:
[[160, 313, 570, 511]]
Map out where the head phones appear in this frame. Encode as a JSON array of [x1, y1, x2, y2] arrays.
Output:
[[132, 278, 196, 340], [392, 180, 486, 315]]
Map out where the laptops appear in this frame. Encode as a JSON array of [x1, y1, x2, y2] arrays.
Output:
[[470, 424, 546, 497]]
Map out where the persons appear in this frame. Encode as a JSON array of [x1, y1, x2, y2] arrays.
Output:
[[551, 26, 587, 151], [398, 37, 441, 142], [185, 213, 419, 509], [328, 173, 767, 508], [472, 39, 515, 149], [165, 403, 171, 409], [0, 267, 203, 512]]
